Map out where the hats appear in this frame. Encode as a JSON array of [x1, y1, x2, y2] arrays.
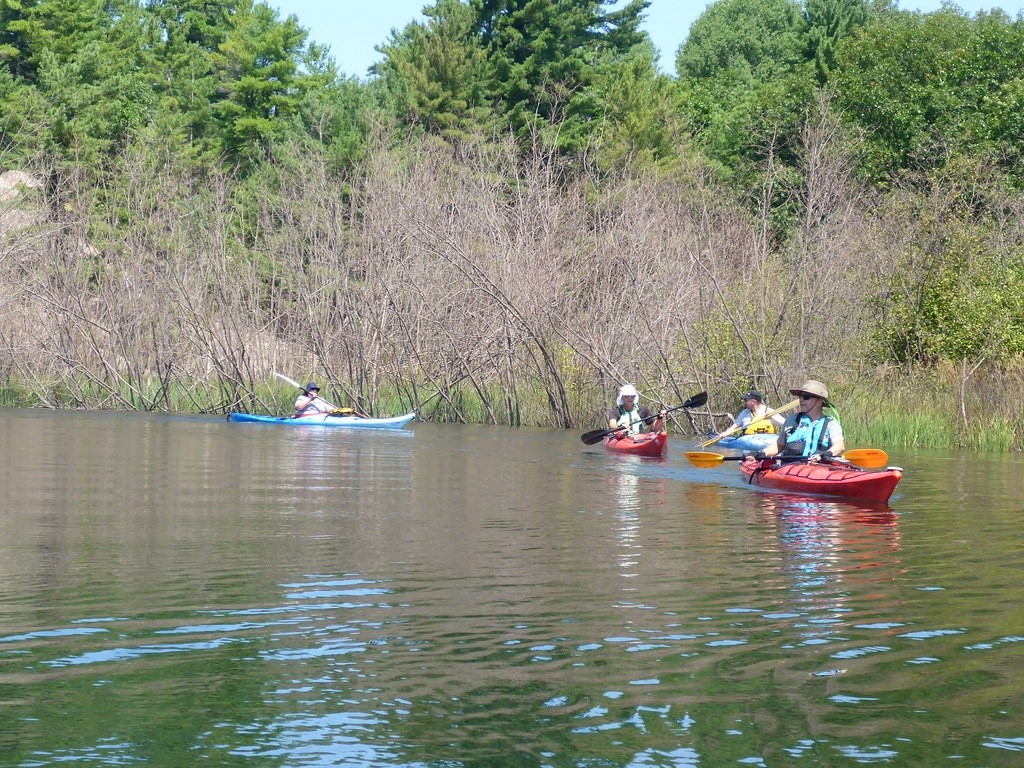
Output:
[[740, 390, 761, 401], [790, 380, 835, 407], [616, 385, 640, 407], [306, 382, 320, 392]]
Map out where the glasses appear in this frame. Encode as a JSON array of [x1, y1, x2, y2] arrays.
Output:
[[623, 396, 635, 398], [799, 394, 820, 400]]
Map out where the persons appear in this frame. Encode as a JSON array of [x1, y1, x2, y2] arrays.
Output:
[[608, 385, 667, 440], [720, 390, 786, 439], [744, 380, 844, 466], [295, 382, 335, 415]]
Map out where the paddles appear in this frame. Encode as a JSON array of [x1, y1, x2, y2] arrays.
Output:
[[580, 391, 710, 445], [273, 370, 356, 417], [683, 448, 891, 469], [700, 399, 801, 447]]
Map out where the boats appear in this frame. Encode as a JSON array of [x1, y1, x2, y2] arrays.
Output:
[[225, 411, 416, 430], [740, 452, 904, 502], [603, 430, 669, 458]]
